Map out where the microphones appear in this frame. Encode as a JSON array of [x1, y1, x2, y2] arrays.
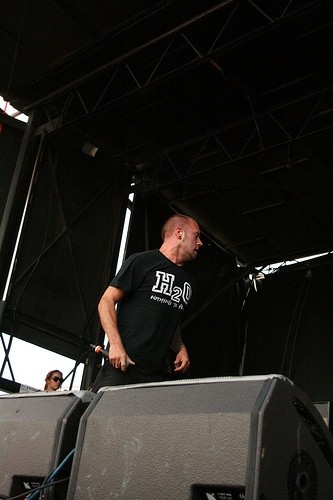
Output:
[[89, 343, 129, 370]]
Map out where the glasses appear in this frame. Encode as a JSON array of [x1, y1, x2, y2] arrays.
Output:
[[48, 377, 64, 383]]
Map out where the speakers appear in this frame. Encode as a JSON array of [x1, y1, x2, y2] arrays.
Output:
[[0, 391, 97, 500], [63, 374, 333, 500]]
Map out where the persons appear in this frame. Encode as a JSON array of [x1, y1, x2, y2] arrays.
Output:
[[92, 215, 202, 393], [43, 370, 63, 392]]
